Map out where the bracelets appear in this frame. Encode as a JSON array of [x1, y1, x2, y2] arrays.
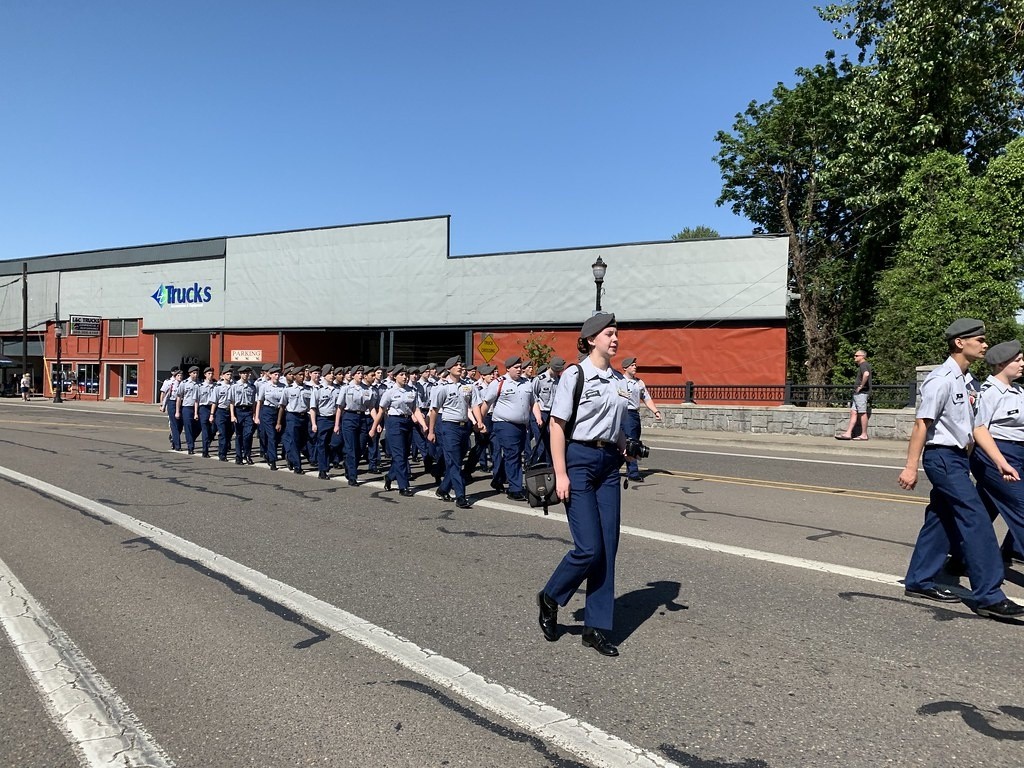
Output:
[[654, 411, 661, 415]]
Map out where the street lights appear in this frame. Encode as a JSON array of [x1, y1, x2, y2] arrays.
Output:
[[53, 325, 64, 404], [592, 255, 607, 311]]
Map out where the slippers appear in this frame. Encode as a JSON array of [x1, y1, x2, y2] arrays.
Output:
[[835, 435, 850, 440], [852, 436, 868, 441]]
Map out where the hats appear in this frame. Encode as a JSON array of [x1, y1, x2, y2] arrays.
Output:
[[985, 340, 1021, 365], [621, 358, 637, 368], [580, 313, 617, 338], [945, 318, 986, 339], [170, 355, 566, 386]]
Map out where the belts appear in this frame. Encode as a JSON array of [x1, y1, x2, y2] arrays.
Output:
[[349, 411, 365, 415], [221, 408, 229, 410], [297, 413, 307, 416], [583, 441, 614, 448], [454, 422, 468, 426], [392, 415, 411, 418], [238, 407, 250, 409]]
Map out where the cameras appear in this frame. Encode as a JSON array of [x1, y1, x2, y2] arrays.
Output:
[[624, 437, 650, 458]]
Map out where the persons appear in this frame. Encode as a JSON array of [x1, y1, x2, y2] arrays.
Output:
[[897, 316, 1024, 619], [536, 313, 642, 656], [20, 373, 31, 402], [834, 350, 873, 441], [159, 356, 662, 509]]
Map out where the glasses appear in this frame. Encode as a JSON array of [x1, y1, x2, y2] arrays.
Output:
[[854, 355, 864, 357]]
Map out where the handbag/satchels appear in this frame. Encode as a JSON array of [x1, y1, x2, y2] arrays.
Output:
[[525, 462, 561, 515]]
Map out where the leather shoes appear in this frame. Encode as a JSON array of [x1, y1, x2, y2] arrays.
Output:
[[537, 590, 559, 641], [628, 475, 644, 482], [977, 598, 1024, 618], [581, 629, 618, 656], [905, 585, 961, 602], [169, 434, 527, 509]]
